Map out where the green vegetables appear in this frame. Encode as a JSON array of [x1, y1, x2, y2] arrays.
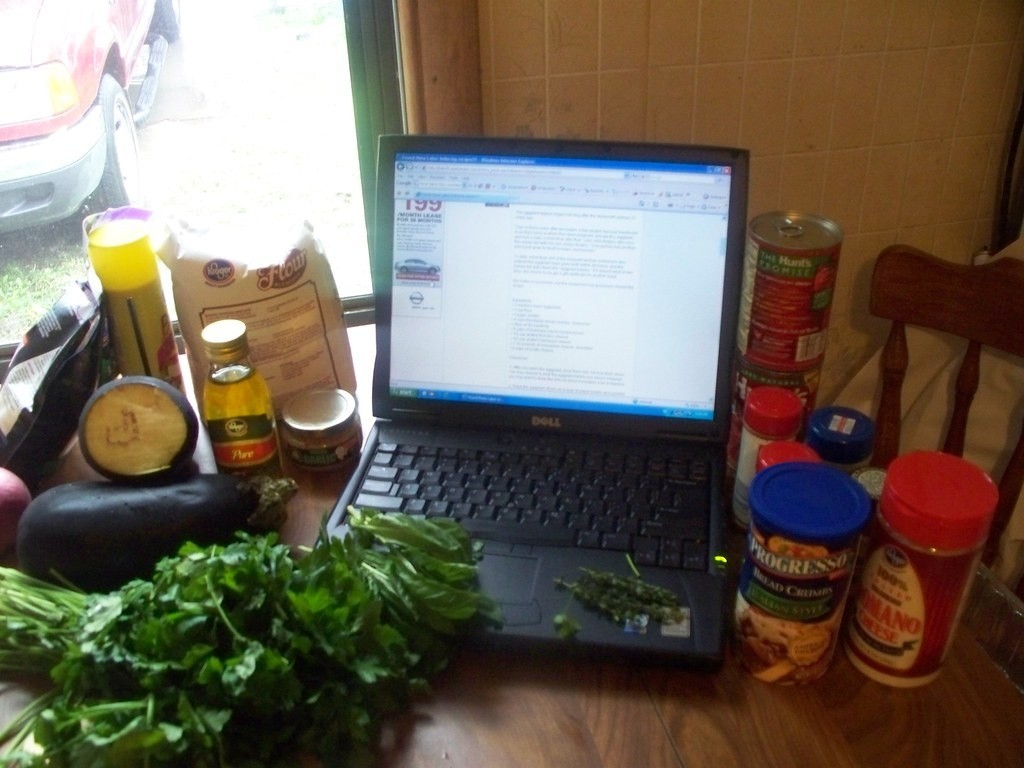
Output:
[[0, 503, 483, 768]]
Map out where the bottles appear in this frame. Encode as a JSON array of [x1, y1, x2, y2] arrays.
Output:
[[201, 318, 279, 472], [87, 217, 184, 391], [280, 385, 364, 470], [729, 384, 1000, 688]]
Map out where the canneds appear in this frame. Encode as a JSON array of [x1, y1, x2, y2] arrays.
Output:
[[723, 207, 997, 689], [280, 387, 362, 471]]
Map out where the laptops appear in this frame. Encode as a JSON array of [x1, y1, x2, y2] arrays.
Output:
[[315, 136, 750, 669]]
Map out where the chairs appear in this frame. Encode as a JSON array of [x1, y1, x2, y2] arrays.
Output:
[[871, 244, 1024, 569]]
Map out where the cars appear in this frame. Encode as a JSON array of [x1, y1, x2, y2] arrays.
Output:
[[0, 0, 181, 243]]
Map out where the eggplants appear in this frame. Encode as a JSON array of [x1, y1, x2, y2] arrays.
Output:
[[14, 473, 297, 594], [77, 376, 199, 484]]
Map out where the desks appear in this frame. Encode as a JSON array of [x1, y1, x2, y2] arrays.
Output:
[[0, 378, 1024, 768]]
